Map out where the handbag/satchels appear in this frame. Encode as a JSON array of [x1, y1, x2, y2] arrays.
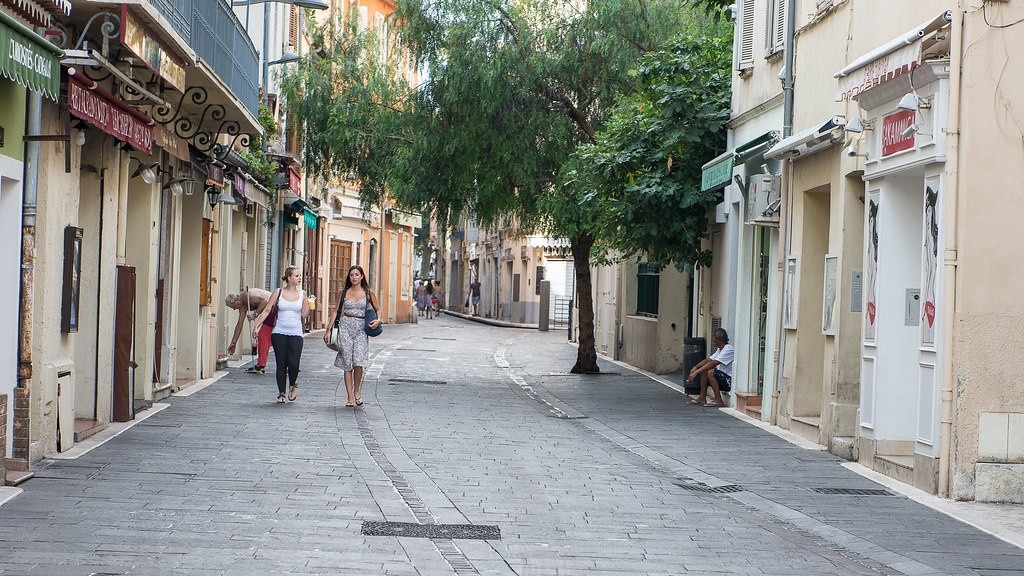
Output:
[[431, 295, 438, 303], [327, 325, 339, 351], [364, 289, 382, 337], [255, 288, 281, 327], [465, 298, 469, 307]]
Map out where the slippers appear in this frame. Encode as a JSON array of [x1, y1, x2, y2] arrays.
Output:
[[345, 402, 354, 407], [355, 397, 363, 405]]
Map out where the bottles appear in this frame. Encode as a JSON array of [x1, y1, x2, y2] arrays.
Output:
[[252, 337, 257, 355]]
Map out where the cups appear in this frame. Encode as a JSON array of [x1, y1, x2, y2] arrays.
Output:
[[308, 298, 316, 309]]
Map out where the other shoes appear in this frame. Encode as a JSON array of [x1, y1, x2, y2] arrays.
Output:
[[244, 365, 265, 375], [288, 382, 298, 401], [277, 392, 285, 403]]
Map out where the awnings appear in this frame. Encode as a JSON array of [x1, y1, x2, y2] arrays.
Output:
[[336, 195, 382, 221], [284, 189, 318, 230], [386, 206, 423, 229], [701, 130, 778, 195]]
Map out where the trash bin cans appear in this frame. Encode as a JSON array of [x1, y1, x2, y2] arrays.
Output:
[[683, 337, 706, 395]]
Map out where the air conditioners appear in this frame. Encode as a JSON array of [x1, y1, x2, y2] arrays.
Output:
[[745, 174, 782, 227]]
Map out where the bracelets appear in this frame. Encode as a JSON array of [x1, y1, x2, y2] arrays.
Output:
[[232, 342, 236, 344], [327, 328, 331, 330], [695, 366, 699, 368]]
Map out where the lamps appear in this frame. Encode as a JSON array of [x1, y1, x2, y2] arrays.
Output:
[[897, 92, 935, 112], [157, 165, 187, 196], [204, 178, 222, 211], [230, 0, 329, 10], [114, 137, 136, 165], [129, 151, 159, 185], [183, 168, 196, 195], [69, 112, 91, 145], [844, 115, 877, 133], [268, 53, 300, 65]]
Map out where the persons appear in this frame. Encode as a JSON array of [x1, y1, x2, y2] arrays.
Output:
[[225, 288, 273, 375], [323, 265, 382, 406], [414, 277, 444, 319], [468, 276, 481, 316], [252, 265, 318, 403], [689, 328, 734, 407]]
